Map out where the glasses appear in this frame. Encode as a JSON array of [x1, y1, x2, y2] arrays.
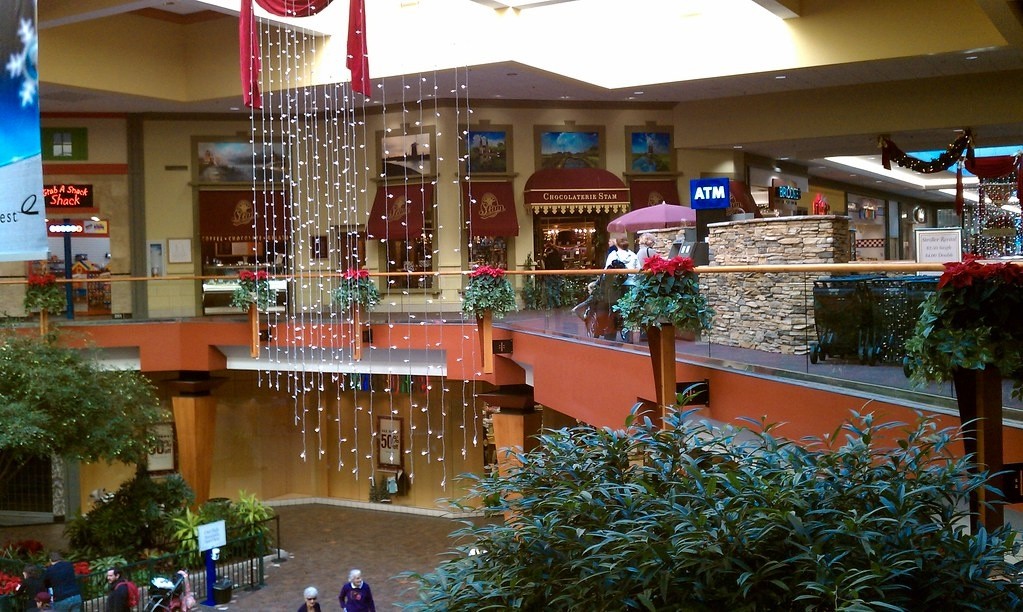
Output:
[[306, 597, 317, 600]]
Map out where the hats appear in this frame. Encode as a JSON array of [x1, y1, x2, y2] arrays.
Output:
[[33, 592, 50, 603]]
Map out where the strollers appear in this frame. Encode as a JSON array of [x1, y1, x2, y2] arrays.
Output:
[[138, 567, 189, 612]]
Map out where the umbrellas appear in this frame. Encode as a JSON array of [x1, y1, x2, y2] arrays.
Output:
[[608, 200, 697, 234]]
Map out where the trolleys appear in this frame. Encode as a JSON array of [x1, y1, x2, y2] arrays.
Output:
[[807, 272, 946, 377]]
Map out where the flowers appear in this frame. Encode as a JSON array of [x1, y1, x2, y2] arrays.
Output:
[[902, 254, 1023, 398], [459, 266, 519, 320], [230, 269, 278, 310], [332, 270, 380, 310], [23, 272, 67, 318], [611, 259, 715, 335]]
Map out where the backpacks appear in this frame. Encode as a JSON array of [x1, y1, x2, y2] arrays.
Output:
[[115, 582, 140, 607]]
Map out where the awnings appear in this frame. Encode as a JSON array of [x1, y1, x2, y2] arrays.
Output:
[[365, 183, 432, 240], [199, 190, 292, 242], [462, 181, 519, 237], [630, 180, 681, 211], [725, 180, 764, 218], [522, 168, 631, 215]]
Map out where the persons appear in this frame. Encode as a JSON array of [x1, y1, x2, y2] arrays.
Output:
[[15, 564, 45, 612], [338, 570, 376, 612], [637, 232, 660, 266], [571, 233, 643, 312], [43, 551, 82, 612], [106, 567, 131, 612], [34, 591, 56, 612], [297, 586, 321, 612], [542, 242, 563, 309]]
